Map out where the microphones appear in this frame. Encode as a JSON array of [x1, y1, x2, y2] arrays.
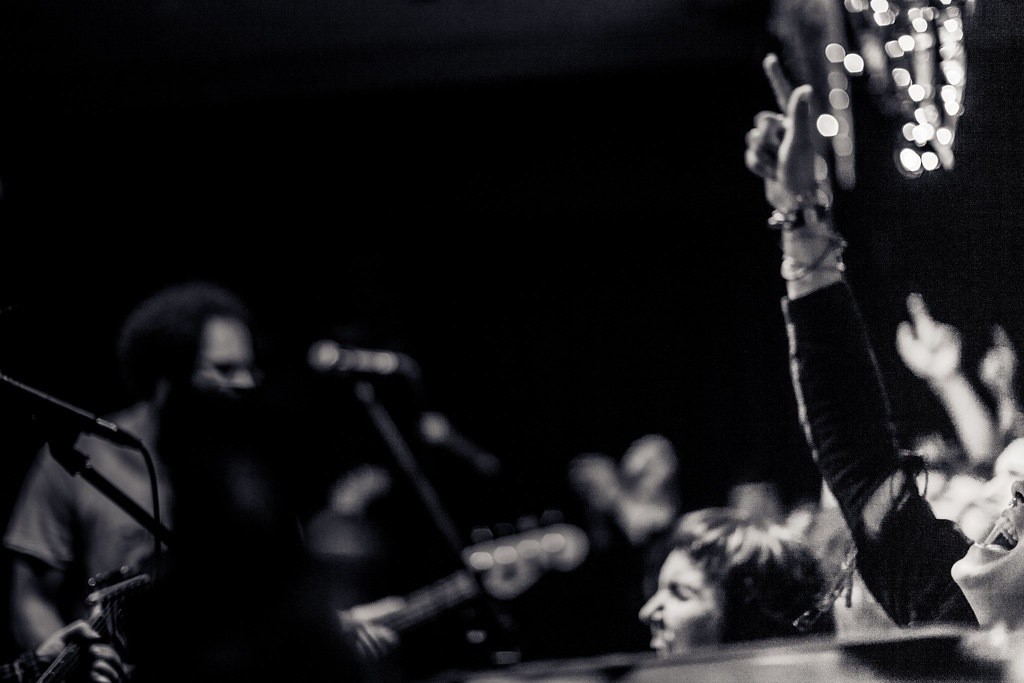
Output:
[[307, 339, 418, 377], [419, 411, 497, 474], [0, 370, 142, 449]]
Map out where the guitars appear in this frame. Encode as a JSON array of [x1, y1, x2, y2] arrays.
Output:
[[341, 525, 588, 647], [29, 567, 151, 683]]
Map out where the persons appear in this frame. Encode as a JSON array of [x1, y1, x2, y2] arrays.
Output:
[[4, 286, 405, 683], [639, 507, 835, 653], [744, 53, 1024, 629]]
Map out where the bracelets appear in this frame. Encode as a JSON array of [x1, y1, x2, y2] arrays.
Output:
[[781, 246, 843, 280], [782, 231, 843, 243], [782, 253, 846, 272], [768, 189, 833, 230]]
